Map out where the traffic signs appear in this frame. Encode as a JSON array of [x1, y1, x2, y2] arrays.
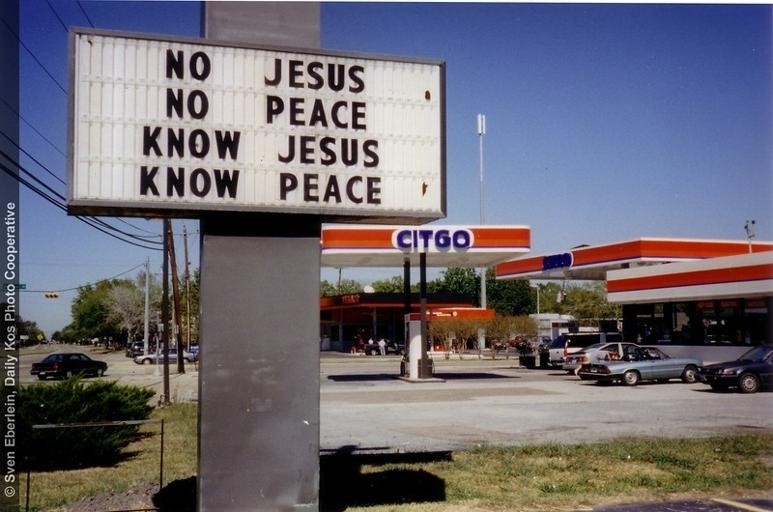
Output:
[[2, 284, 27, 289]]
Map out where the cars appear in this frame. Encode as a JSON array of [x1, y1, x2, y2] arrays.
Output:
[[125, 337, 199, 366], [578, 346, 704, 386], [365, 337, 404, 356], [562, 341, 642, 373], [31, 352, 108, 381], [693, 345, 772, 393]]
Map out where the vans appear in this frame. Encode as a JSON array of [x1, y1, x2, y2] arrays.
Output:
[[547, 331, 624, 366]]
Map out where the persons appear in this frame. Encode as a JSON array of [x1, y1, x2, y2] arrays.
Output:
[[94, 336, 109, 350], [350, 333, 386, 357]]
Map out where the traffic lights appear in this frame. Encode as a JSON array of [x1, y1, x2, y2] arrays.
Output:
[[45, 292, 59, 300]]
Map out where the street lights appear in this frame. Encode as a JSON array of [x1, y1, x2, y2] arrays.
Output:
[[476, 112, 488, 309]]
